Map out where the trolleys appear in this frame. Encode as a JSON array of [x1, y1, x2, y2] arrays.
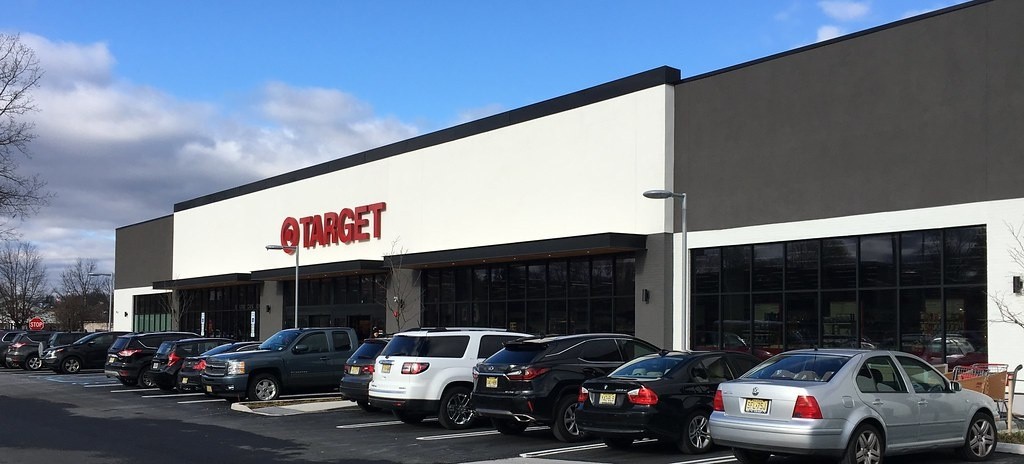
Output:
[[953, 360, 1014, 422]]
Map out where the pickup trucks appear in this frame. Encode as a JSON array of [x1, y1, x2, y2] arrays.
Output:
[[200, 327, 362, 402]]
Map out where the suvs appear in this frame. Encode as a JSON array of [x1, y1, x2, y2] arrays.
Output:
[[468, 334, 663, 445], [0, 329, 27, 370], [38, 329, 96, 373], [6, 331, 57, 372], [340, 333, 397, 413], [150, 337, 238, 394], [103, 331, 206, 390], [369, 327, 559, 431]]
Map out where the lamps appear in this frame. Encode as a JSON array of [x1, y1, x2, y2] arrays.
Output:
[[1012, 276, 1023, 294], [124, 312, 128, 317], [265, 305, 270, 312], [642, 288, 649, 301]]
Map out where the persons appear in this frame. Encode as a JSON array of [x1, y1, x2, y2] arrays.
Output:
[[372, 327, 379, 338]]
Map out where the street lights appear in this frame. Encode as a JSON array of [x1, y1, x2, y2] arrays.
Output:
[[264, 244, 299, 328], [643, 189, 688, 352], [88, 272, 113, 331]]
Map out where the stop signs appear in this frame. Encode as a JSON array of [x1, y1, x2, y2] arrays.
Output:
[[29, 317, 44, 332]]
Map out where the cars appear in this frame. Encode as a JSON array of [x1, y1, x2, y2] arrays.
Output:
[[691, 319, 988, 361], [573, 350, 764, 455], [176, 339, 264, 389], [709, 349, 1000, 464], [39, 330, 142, 375], [196, 328, 233, 339]]
